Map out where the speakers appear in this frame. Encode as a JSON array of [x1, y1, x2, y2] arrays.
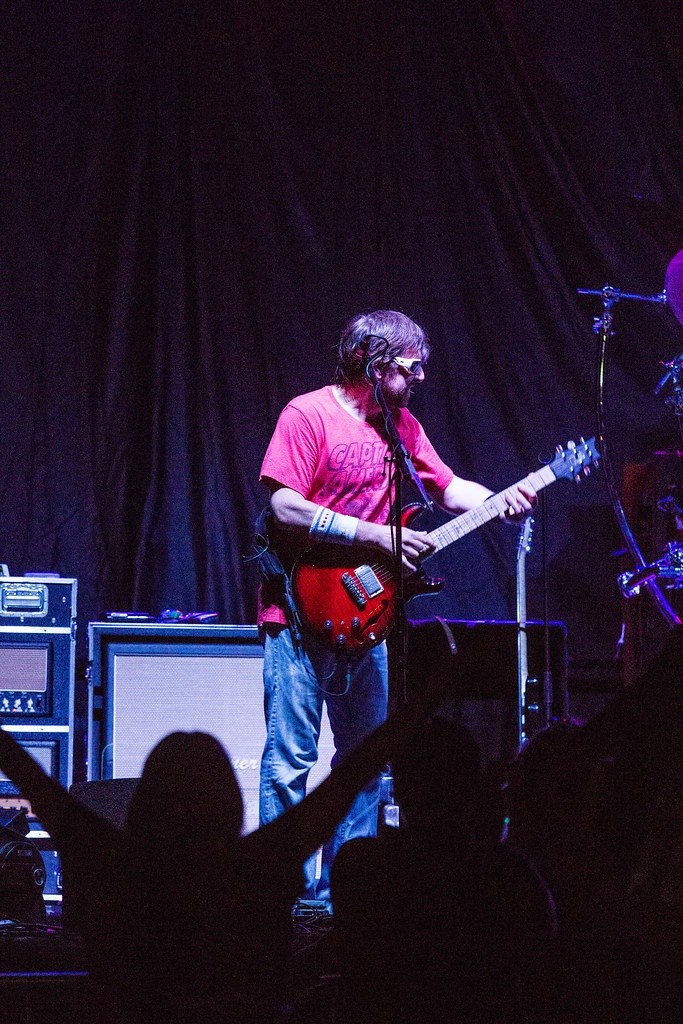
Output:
[[0, 566, 339, 902]]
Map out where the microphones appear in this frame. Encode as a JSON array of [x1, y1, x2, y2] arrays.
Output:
[[348, 337, 371, 365]]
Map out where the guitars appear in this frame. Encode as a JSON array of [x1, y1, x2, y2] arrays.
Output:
[[287, 434, 601, 659]]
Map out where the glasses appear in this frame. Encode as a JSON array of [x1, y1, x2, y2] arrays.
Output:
[[392, 357, 422, 372]]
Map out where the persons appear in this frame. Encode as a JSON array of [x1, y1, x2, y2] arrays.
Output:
[[245, 311, 539, 915], [507, 719, 622, 915], [0, 649, 474, 1024], [290, 714, 559, 1024]]
